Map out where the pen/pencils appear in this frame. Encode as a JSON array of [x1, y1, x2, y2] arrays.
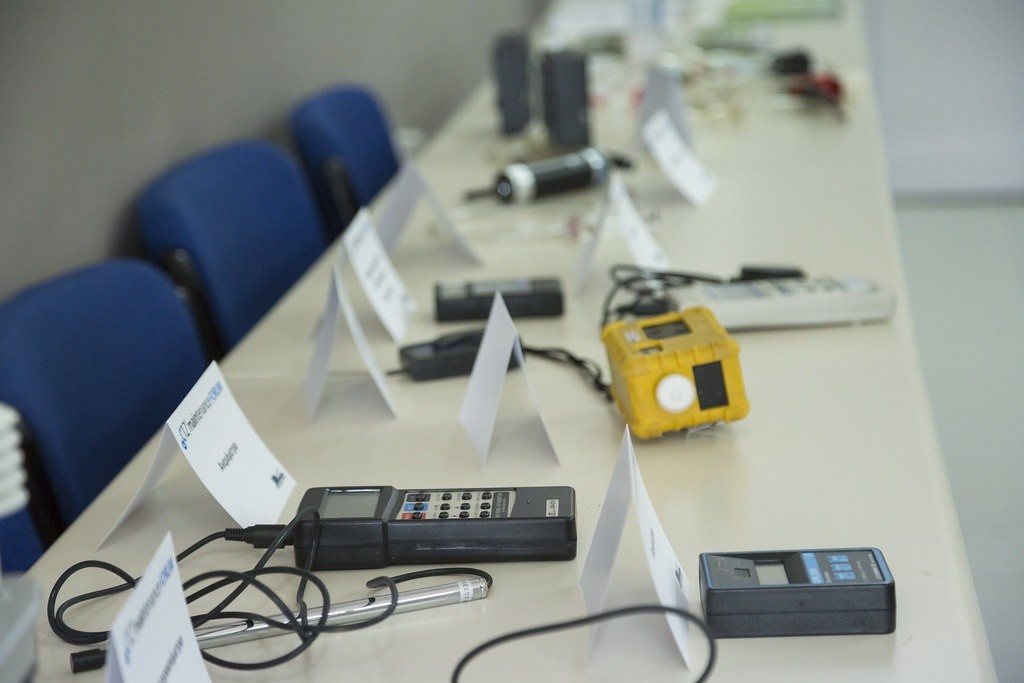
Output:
[[69, 577, 487, 672]]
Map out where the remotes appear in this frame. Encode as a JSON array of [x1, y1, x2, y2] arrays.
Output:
[[666, 270, 896, 330]]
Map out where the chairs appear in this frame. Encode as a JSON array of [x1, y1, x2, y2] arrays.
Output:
[[293, 84, 398, 236], [0, 257, 209, 545], [138, 144, 324, 360]]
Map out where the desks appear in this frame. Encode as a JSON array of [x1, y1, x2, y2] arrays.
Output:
[[24, 0, 995, 683]]
